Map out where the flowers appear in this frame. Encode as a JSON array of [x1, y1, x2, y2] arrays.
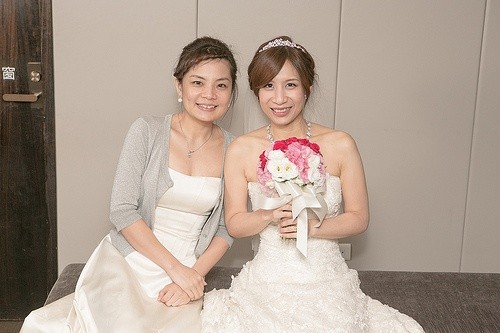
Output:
[[257, 138, 329, 198]]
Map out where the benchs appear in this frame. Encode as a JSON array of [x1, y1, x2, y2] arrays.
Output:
[[44, 263, 500, 333]]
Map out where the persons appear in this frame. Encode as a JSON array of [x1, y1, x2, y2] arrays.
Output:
[[224, 36, 370, 333], [73, 37, 237, 333]]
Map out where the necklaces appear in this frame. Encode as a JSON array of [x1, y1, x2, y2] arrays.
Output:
[[178, 112, 213, 156], [265, 120, 312, 144]]
